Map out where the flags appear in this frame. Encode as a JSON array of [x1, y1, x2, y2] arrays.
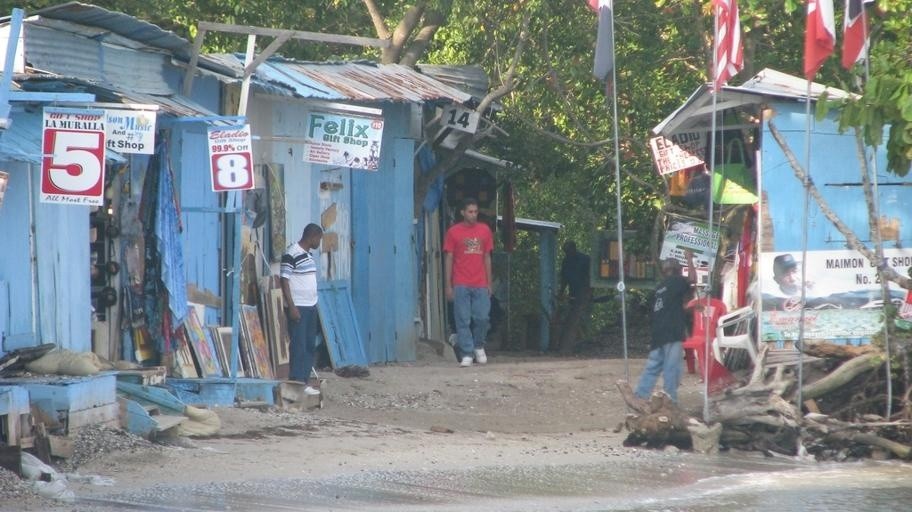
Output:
[[586, 0, 614, 102], [802, 0, 837, 80], [842, 1, 870, 70], [708, 1, 745, 93]]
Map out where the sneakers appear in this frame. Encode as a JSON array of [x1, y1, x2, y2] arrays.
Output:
[[472, 347, 488, 364], [459, 356, 474, 368], [302, 386, 320, 396]]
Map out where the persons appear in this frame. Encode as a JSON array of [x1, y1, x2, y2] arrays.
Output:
[[442, 197, 494, 368], [772, 253, 801, 294], [559, 239, 595, 337], [279, 221, 324, 397], [633, 247, 697, 402]]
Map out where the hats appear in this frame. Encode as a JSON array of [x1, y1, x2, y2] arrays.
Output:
[[770, 252, 803, 280]]
